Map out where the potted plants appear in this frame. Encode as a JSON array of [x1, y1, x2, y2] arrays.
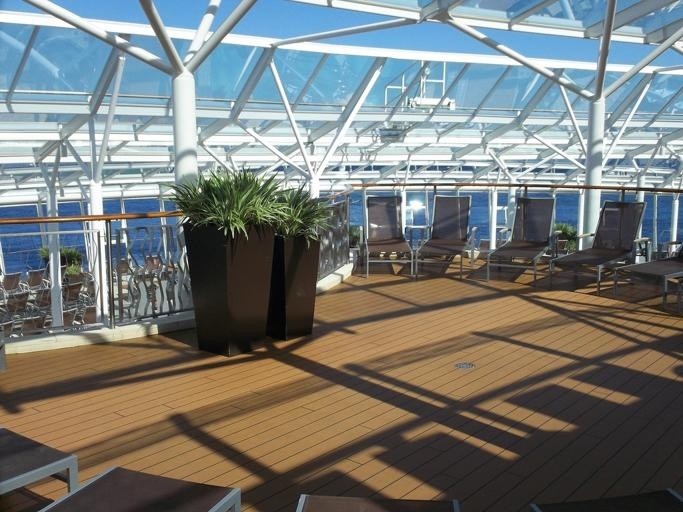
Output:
[[154, 163, 347, 358]]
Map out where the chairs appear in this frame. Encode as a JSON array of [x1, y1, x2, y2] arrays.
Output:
[[361, 194, 413, 279], [0, 254, 178, 339], [487, 197, 563, 287], [613, 241, 683, 312], [415, 195, 478, 281], [549, 200, 652, 296]]
